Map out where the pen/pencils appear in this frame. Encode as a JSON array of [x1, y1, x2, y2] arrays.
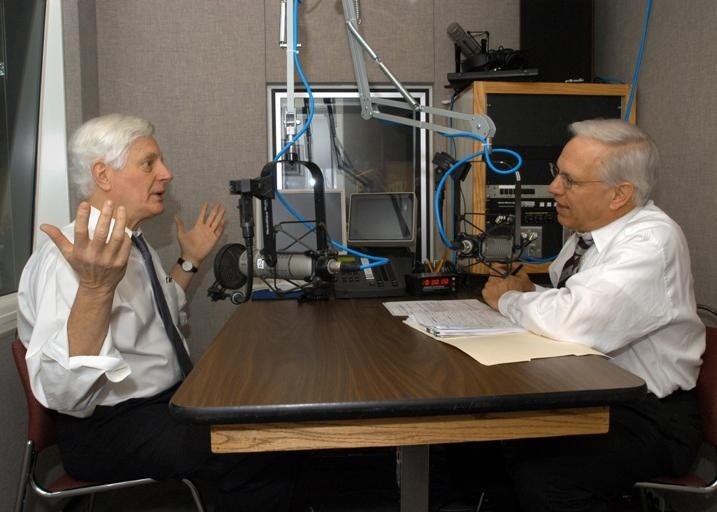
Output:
[[425, 258, 444, 273], [511, 263, 523, 275]]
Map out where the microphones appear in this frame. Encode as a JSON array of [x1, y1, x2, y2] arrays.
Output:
[[459, 235, 515, 262], [446, 22, 482, 58], [213, 242, 340, 292]]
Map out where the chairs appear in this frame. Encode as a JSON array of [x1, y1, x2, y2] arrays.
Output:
[[8, 338, 205, 511], [630, 303, 716, 511]]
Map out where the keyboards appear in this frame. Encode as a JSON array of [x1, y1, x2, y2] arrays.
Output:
[[332, 251, 406, 299]]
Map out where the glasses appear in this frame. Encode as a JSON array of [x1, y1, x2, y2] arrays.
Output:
[[545, 159, 619, 189]]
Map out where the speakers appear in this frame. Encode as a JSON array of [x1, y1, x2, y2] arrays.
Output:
[[519, 0, 595, 83]]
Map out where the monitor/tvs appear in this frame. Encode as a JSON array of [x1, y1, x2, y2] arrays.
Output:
[[347, 190, 418, 253]]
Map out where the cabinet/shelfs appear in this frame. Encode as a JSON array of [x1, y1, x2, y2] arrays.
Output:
[[442, 80, 637, 275]]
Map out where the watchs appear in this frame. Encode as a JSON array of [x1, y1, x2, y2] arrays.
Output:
[[178, 257, 198, 274]]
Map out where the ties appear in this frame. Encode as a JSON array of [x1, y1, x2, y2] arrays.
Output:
[[127, 229, 200, 384], [555, 236, 599, 291]]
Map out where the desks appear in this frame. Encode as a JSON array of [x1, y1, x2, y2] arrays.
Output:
[[167, 280, 647, 512]]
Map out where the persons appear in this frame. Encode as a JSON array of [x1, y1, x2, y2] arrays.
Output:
[[16, 114, 263, 511], [481, 117, 708, 511]]
